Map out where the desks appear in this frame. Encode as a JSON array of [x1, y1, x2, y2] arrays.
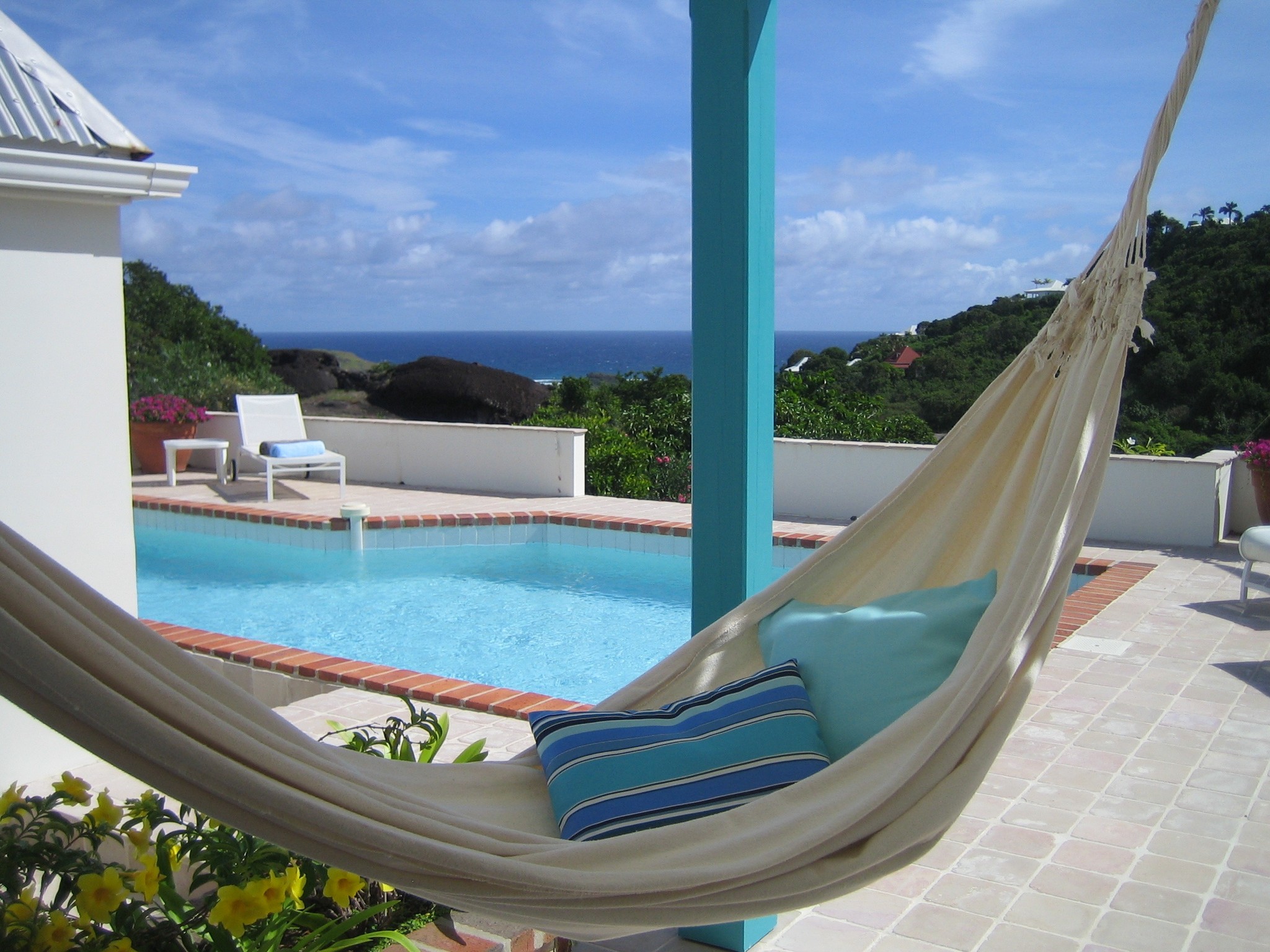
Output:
[[163, 438, 230, 486]]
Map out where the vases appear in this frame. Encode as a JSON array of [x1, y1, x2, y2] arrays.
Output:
[[131, 421, 196, 473], [1249, 468, 1270, 525]]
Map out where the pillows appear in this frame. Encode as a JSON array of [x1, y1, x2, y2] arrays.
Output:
[[756, 567, 996, 767], [260, 438, 324, 457], [528, 660, 830, 846]]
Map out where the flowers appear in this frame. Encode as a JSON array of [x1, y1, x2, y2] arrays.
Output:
[[130, 393, 212, 425], [1236, 438, 1270, 470]]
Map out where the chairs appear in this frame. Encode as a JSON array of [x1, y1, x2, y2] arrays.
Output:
[[1240, 525, 1270, 602], [237, 393, 344, 503]]
